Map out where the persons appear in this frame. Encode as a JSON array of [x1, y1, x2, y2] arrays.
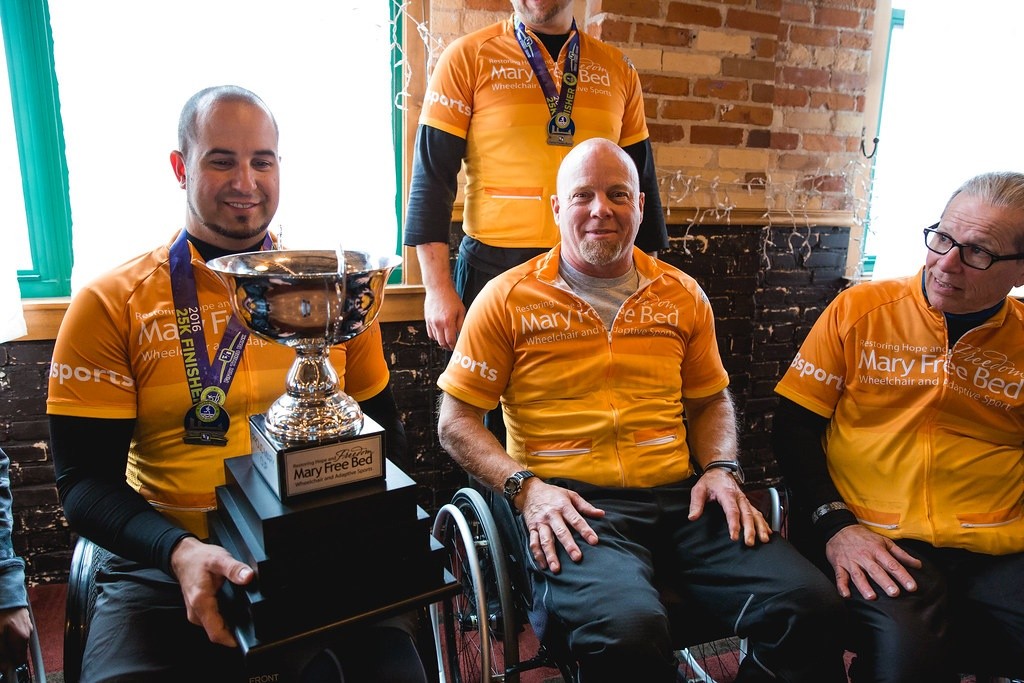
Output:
[[773, 173, 1024, 683], [47, 85, 441, 683], [404, 1, 670, 640], [436, 137, 851, 683], [0, 445, 34, 651]]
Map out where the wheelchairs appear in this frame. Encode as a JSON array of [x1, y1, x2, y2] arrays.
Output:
[[427, 404, 787, 683], [64, 534, 444, 682]]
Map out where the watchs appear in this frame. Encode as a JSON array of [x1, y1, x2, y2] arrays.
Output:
[[703, 459, 745, 490], [503, 469, 535, 512]]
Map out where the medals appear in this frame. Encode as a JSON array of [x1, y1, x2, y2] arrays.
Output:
[[182, 402, 231, 448], [545, 114, 576, 148]]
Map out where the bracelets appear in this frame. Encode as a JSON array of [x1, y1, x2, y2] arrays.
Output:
[[810, 502, 851, 527]]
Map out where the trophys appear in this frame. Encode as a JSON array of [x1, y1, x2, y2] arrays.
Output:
[[204, 251, 465, 666]]
